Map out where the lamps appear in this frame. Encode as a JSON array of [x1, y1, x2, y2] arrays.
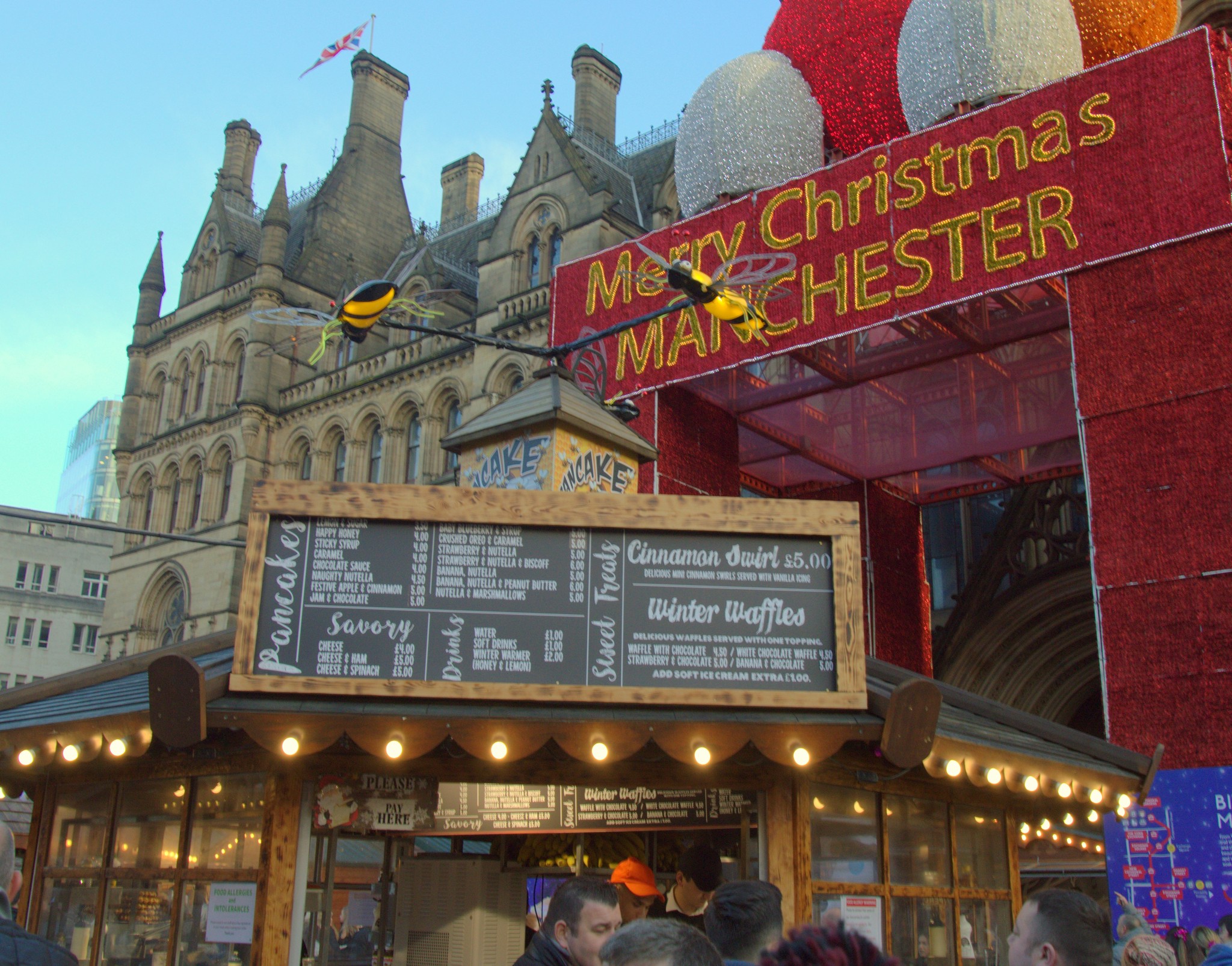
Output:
[[1017, 773, 1038, 792], [978, 765, 1002, 785], [18, 745, 43, 765], [491, 734, 507, 760], [1113, 792, 1132, 809], [692, 741, 711, 765], [62, 741, 87, 762], [1049, 779, 1072, 799], [936, 757, 962, 777], [282, 730, 302, 756], [1082, 786, 1103, 803], [591, 737, 609, 761], [109, 734, 135, 757], [386, 733, 404, 759], [789, 740, 810, 766]]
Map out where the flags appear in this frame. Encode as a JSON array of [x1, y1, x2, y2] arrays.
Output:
[[298, 19, 371, 78]]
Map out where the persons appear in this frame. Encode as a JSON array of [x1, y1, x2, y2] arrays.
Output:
[[302, 903, 376, 966], [1, 818, 81, 966], [508, 875, 623, 965], [607, 855, 665, 928], [595, 916, 725, 966], [1109, 890, 1232, 966], [1006, 888, 1114, 965], [758, 920, 903, 966], [703, 880, 785, 965], [645, 844, 726, 938], [910, 932, 934, 966]]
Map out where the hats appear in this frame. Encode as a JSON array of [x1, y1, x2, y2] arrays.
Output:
[[611, 857, 666, 904], [679, 843, 722, 893]]
